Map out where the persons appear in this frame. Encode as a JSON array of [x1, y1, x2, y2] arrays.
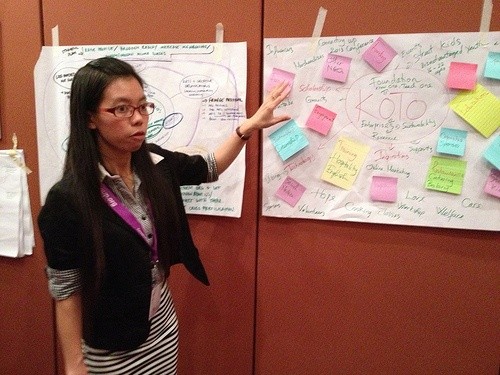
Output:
[[36, 57, 296, 375]]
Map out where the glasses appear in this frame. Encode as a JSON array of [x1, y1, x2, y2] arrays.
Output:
[[97, 102, 156, 118]]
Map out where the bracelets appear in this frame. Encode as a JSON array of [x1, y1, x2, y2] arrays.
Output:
[[236, 127, 252, 140]]
